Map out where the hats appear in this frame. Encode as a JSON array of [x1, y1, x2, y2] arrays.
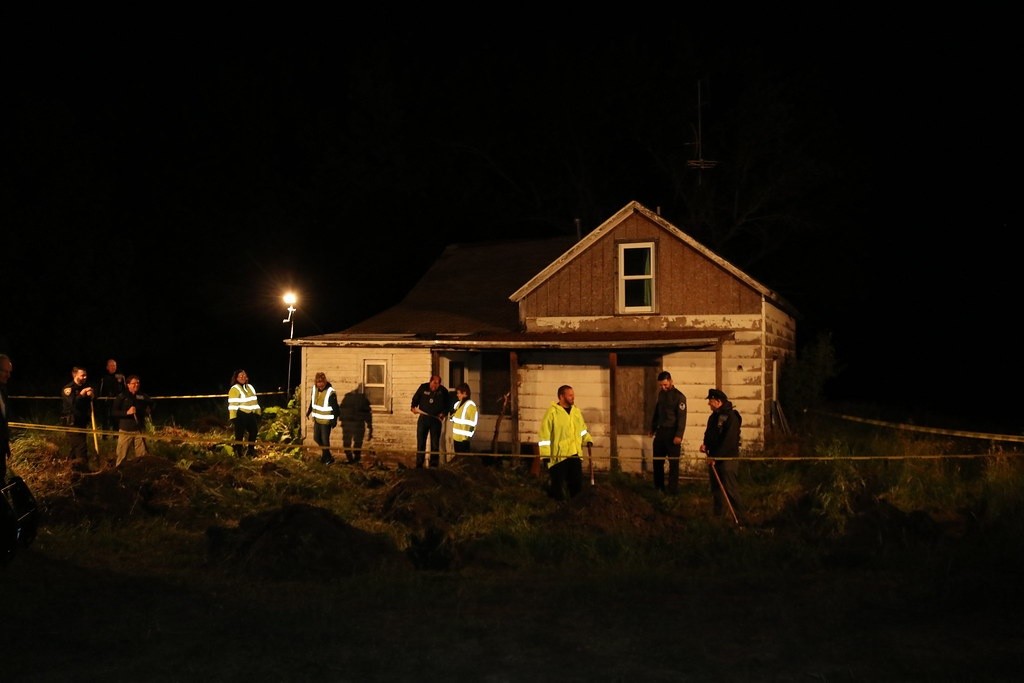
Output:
[[705, 389, 727, 401]]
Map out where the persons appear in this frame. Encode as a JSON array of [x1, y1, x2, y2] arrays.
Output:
[[111, 375, 148, 467], [448, 383, 478, 453], [0, 354, 13, 482], [411, 375, 451, 469], [699, 389, 742, 516], [538, 385, 594, 506], [649, 371, 687, 494], [95, 359, 126, 441], [227, 369, 261, 457], [62, 367, 95, 463], [306, 373, 339, 464]]
